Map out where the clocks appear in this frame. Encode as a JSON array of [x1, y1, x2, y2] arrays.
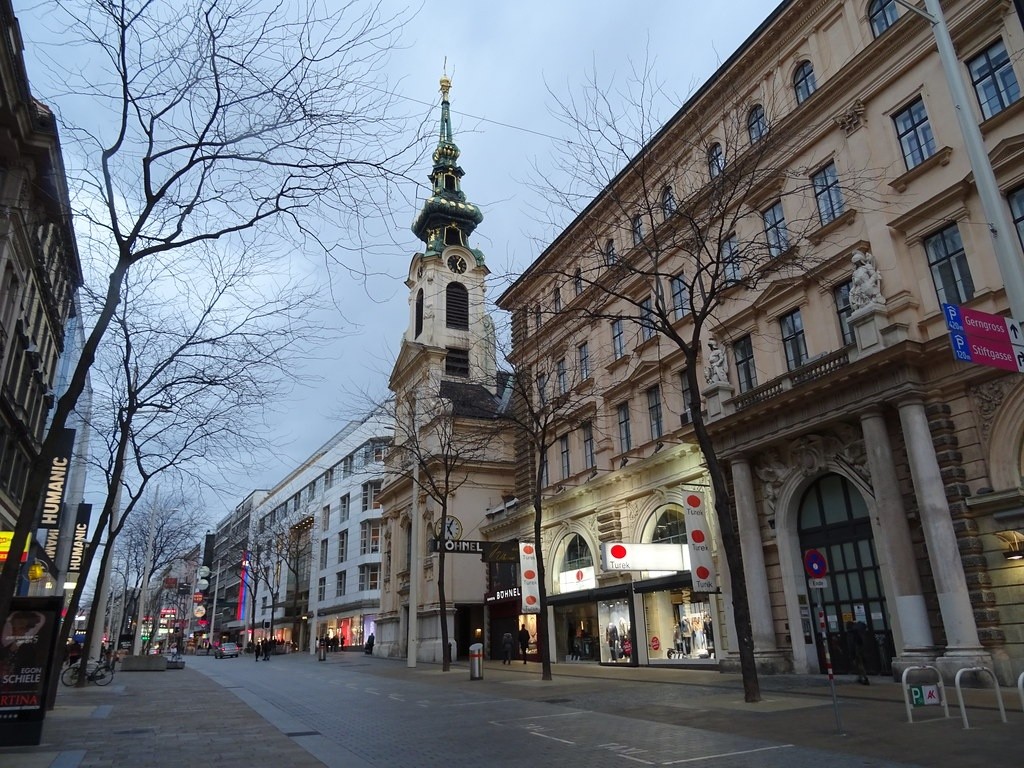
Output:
[[447, 255, 467, 274], [416, 264, 423, 278]]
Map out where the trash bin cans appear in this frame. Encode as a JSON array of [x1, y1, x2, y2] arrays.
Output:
[[319, 644, 327, 661], [468, 642, 484, 680]]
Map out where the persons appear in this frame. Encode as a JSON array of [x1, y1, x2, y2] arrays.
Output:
[[517, 624, 530, 664], [704, 338, 729, 385], [325, 634, 330, 653], [846, 620, 870, 685], [331, 635, 339, 652], [143, 639, 179, 662], [502, 630, 514, 665], [63, 642, 133, 672], [320, 635, 325, 646], [255, 642, 261, 661], [849, 249, 886, 310], [262, 637, 298, 661], [605, 619, 632, 663], [341, 634, 345, 651], [673, 612, 716, 659], [367, 633, 374, 654], [197, 634, 252, 656]]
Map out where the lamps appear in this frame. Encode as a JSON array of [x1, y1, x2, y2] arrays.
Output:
[[1002, 542, 1024, 560]]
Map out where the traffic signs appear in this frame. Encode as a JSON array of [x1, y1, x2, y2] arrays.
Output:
[[941, 303, 1024, 374]]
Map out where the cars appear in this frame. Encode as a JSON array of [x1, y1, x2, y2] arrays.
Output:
[[214, 642, 239, 659]]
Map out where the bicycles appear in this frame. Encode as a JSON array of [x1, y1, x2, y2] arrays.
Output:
[[61, 655, 113, 687]]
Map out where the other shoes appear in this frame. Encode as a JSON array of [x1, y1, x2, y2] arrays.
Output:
[[855, 676, 871, 686], [508, 660, 511, 665], [262, 659, 266, 661], [502, 660, 507, 665], [267, 658, 269, 660], [523, 660, 527, 664], [256, 660, 259, 662]]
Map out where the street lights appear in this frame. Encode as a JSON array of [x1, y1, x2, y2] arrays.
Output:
[[132, 485, 180, 655], [199, 560, 221, 648]]
